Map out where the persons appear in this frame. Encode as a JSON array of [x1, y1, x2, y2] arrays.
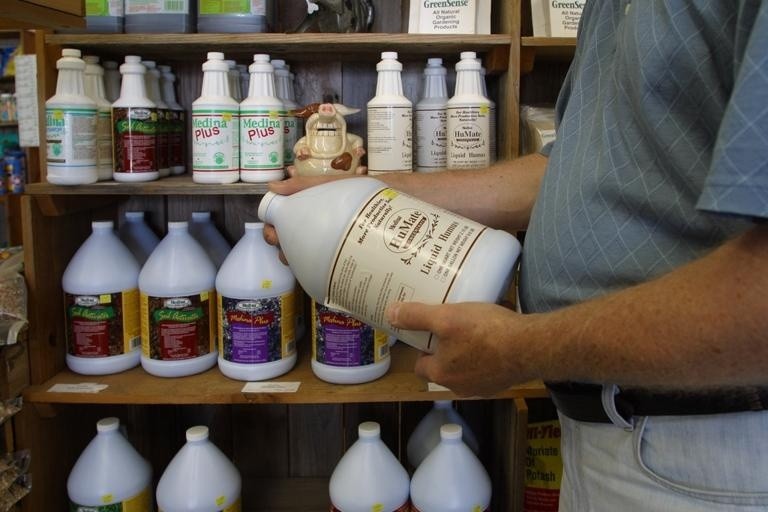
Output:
[[258, 0, 767, 510]]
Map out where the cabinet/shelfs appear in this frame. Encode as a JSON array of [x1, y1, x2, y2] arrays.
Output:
[[0, 0, 578, 512]]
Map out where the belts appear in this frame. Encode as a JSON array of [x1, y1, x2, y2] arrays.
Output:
[[543, 381, 768, 423]]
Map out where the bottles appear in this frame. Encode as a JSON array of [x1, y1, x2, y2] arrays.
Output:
[[41, 48, 299, 186], [364, 50, 496, 180]]
